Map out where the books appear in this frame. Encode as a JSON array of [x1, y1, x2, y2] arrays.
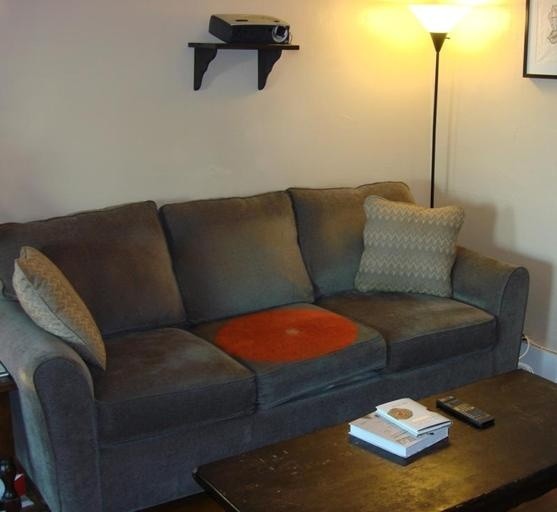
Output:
[[346, 396, 454, 467]]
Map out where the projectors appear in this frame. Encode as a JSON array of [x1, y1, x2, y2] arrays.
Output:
[[208, 14, 290, 44]]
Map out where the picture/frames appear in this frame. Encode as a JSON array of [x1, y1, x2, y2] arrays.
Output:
[[523, 0, 557, 79]]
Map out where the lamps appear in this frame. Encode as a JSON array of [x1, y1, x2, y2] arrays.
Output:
[[412, 5, 471, 208]]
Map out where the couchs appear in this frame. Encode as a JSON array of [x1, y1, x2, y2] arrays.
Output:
[[0, 247, 530, 512]]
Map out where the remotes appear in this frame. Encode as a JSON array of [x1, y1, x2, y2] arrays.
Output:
[[437, 395, 495, 428]]
